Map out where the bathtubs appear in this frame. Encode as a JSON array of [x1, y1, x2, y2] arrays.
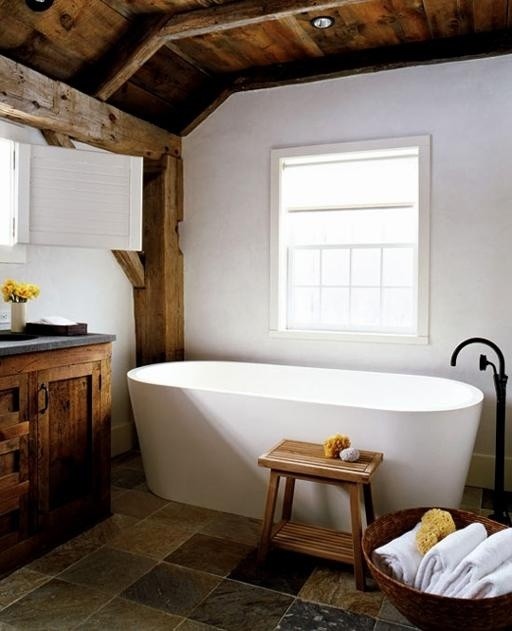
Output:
[[126, 361, 485, 535]]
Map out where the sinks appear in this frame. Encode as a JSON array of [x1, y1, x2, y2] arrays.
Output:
[[0, 333, 39, 342]]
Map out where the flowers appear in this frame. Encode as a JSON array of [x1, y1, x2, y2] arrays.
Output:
[[0, 278, 43, 304]]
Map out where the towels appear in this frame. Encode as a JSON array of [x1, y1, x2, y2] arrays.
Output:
[[371, 517, 512, 599]]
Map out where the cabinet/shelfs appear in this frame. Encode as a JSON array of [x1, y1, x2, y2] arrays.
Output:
[[0, 341, 114, 582]]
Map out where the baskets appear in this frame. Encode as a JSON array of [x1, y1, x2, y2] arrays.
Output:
[[361, 507, 512, 630]]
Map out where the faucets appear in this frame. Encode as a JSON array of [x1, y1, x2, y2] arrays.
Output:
[[450, 338, 505, 375]]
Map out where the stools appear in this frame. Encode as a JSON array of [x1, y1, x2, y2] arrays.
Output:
[[255, 439, 384, 594]]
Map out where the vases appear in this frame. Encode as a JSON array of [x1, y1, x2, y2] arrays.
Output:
[[10, 300, 28, 333]]
